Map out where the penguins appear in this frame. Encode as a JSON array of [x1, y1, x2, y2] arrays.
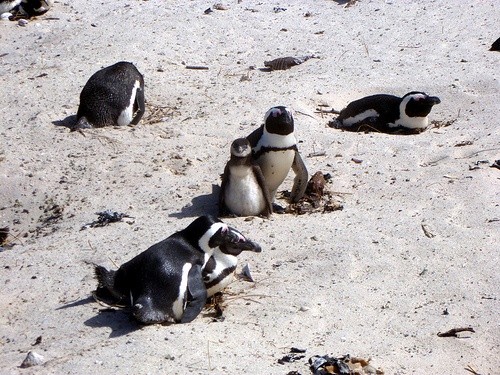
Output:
[[69, 61, 145, 131], [217, 137, 273, 219], [336, 91, 441, 135], [91, 215, 241, 324], [201, 226, 262, 298], [0, 0, 56, 21], [246, 106, 309, 205]]
